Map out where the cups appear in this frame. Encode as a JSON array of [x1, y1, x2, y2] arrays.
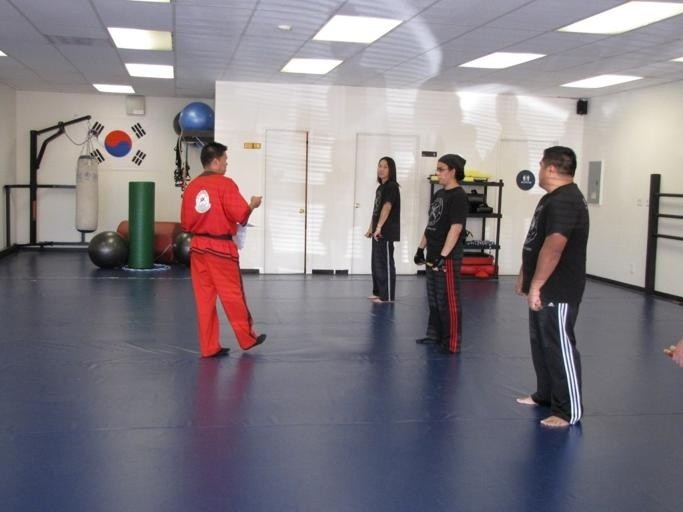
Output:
[[435, 166, 450, 172]]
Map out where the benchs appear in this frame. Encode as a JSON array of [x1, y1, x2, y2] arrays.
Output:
[[83, 115, 152, 170]]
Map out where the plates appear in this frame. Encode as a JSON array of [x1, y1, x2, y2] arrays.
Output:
[[429, 176, 503, 281]]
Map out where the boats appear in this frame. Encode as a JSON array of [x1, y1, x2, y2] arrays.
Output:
[[577, 101, 587, 114]]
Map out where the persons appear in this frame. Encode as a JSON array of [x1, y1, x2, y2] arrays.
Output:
[[414, 153, 470, 355], [364, 156, 400, 303], [181, 143, 267, 358], [515, 147, 589, 427]]
